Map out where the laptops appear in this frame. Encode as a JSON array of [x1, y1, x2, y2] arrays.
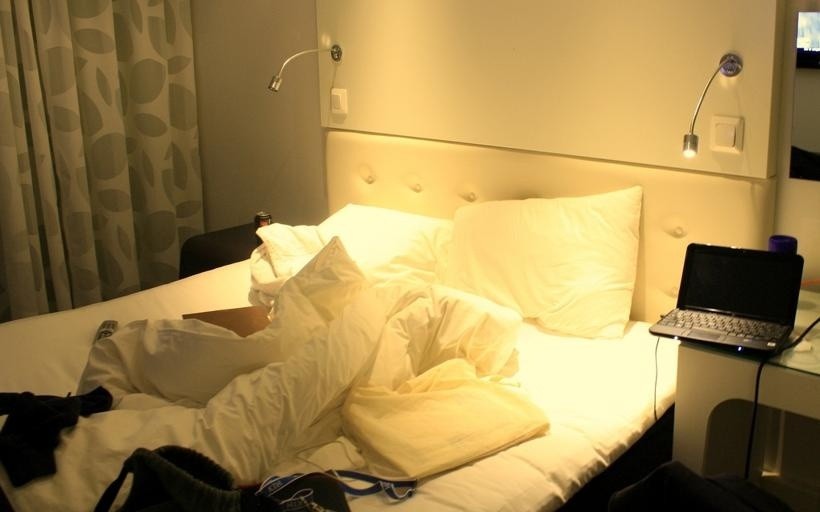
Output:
[[647, 240, 805, 358]]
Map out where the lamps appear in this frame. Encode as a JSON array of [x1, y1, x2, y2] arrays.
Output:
[[682, 53, 743, 159], [268, 42, 343, 95]]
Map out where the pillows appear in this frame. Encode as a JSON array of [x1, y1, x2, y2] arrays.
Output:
[[434, 187, 643, 342]]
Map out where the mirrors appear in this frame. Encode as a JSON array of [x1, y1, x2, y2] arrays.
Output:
[[787, 10, 819, 183]]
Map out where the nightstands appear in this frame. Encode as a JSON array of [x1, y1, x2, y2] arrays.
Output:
[[179, 221, 260, 279]]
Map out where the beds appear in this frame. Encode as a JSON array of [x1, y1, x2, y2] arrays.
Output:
[[0, 205, 681, 512]]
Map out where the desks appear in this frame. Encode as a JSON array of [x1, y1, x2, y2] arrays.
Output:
[[673, 290, 820, 481]]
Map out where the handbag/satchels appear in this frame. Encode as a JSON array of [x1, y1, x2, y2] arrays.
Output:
[[120, 445, 240, 511], [241, 471, 351, 512]]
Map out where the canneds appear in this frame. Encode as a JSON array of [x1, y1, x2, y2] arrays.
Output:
[[255, 212, 273, 246]]
